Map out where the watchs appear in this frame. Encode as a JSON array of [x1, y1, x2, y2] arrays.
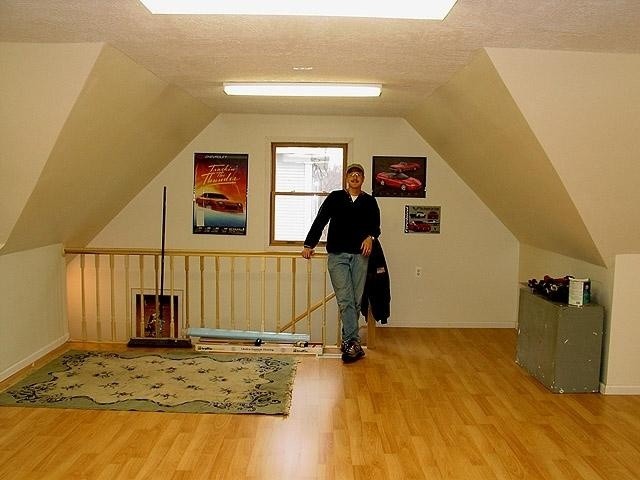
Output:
[[368, 235, 375, 242]]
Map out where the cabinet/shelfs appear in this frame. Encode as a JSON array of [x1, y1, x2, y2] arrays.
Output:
[[516, 285, 604, 395]]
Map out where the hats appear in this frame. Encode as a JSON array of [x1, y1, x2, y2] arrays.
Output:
[[345, 164, 364, 174]]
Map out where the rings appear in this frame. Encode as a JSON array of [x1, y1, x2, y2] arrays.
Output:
[[369, 250, 371, 253]]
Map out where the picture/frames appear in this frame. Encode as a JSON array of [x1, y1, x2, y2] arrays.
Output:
[[130, 288, 184, 339], [192, 153, 248, 236]]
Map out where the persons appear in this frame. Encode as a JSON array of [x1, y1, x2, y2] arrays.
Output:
[[301, 164, 381, 363]]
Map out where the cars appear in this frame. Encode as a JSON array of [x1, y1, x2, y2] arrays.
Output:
[[408, 209, 439, 233], [195, 191, 244, 214]]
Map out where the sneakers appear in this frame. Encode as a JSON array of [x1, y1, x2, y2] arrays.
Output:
[[342, 341, 365, 362], [340, 338, 365, 355]]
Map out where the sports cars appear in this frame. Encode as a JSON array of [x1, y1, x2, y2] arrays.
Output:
[[388, 161, 421, 172], [376, 171, 422, 193]]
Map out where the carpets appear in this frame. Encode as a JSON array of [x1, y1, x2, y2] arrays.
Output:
[[1, 348, 298, 418]]
